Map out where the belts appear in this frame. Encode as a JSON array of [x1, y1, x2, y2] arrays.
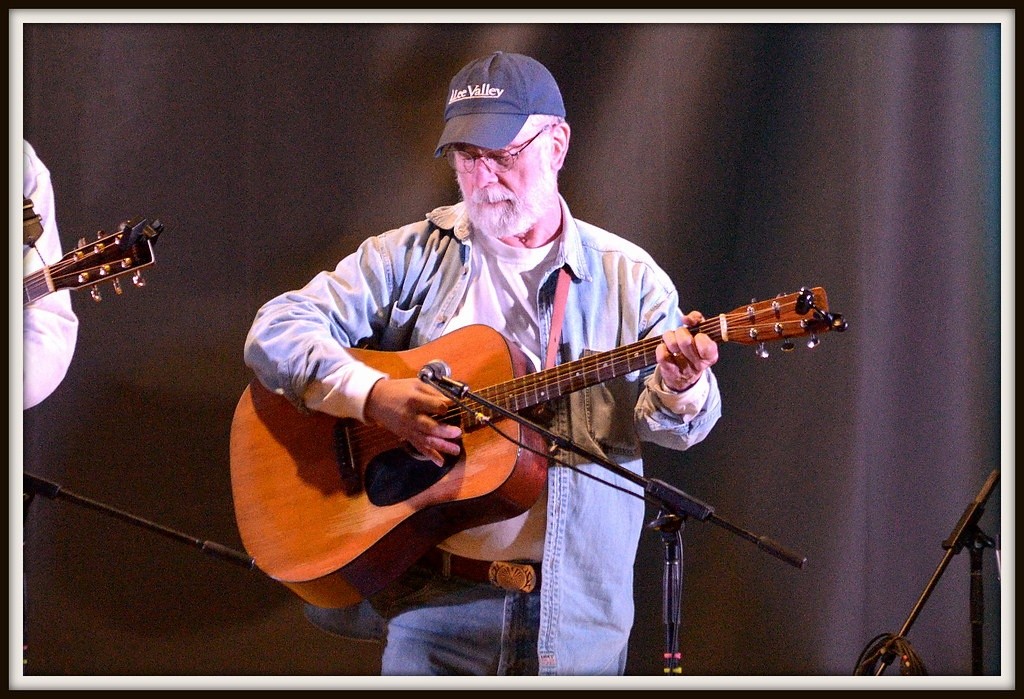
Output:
[[426, 545, 549, 591]]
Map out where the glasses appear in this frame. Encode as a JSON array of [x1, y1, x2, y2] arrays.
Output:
[[443, 115, 563, 175]]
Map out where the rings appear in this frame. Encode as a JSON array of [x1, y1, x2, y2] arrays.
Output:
[[673, 351, 682, 357]]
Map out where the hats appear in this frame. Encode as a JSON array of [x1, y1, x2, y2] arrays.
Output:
[[432, 49, 567, 149]]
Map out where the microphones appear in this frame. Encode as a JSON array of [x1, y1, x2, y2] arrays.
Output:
[[419, 360, 451, 382]]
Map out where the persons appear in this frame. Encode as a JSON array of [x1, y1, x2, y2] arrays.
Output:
[[242, 50, 722, 676], [23, 138, 79, 411]]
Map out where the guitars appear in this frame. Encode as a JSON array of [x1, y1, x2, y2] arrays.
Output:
[[24, 214, 166, 309], [227, 286, 851, 608]]
